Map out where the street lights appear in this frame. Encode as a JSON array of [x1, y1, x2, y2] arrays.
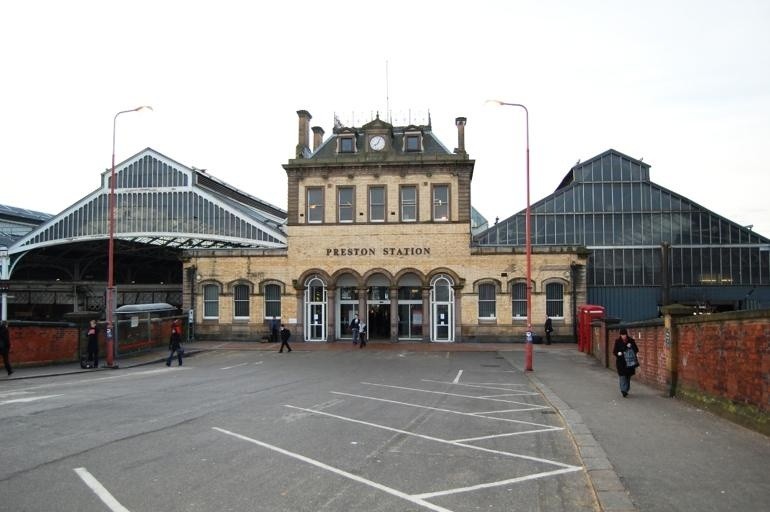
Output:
[[485, 98, 536, 372], [106, 106, 151, 368]]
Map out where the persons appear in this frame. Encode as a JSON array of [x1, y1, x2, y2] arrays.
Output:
[[270, 315, 280, 341], [279, 324, 292, 353], [544, 314, 553, 344], [349, 313, 360, 345], [613, 327, 639, 397], [0, 320, 15, 376], [87, 317, 100, 369], [165, 327, 182, 368], [357, 320, 368, 348], [168, 317, 183, 352]]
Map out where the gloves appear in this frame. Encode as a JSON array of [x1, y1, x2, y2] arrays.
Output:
[[627, 343, 631, 347], [618, 352, 622, 356]]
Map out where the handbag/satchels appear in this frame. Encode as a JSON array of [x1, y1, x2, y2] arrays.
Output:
[[624, 350, 639, 368]]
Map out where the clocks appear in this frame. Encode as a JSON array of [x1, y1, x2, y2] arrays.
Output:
[[369, 135, 386, 152]]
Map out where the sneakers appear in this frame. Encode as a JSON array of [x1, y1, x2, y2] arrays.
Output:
[[623, 392, 627, 397]]
